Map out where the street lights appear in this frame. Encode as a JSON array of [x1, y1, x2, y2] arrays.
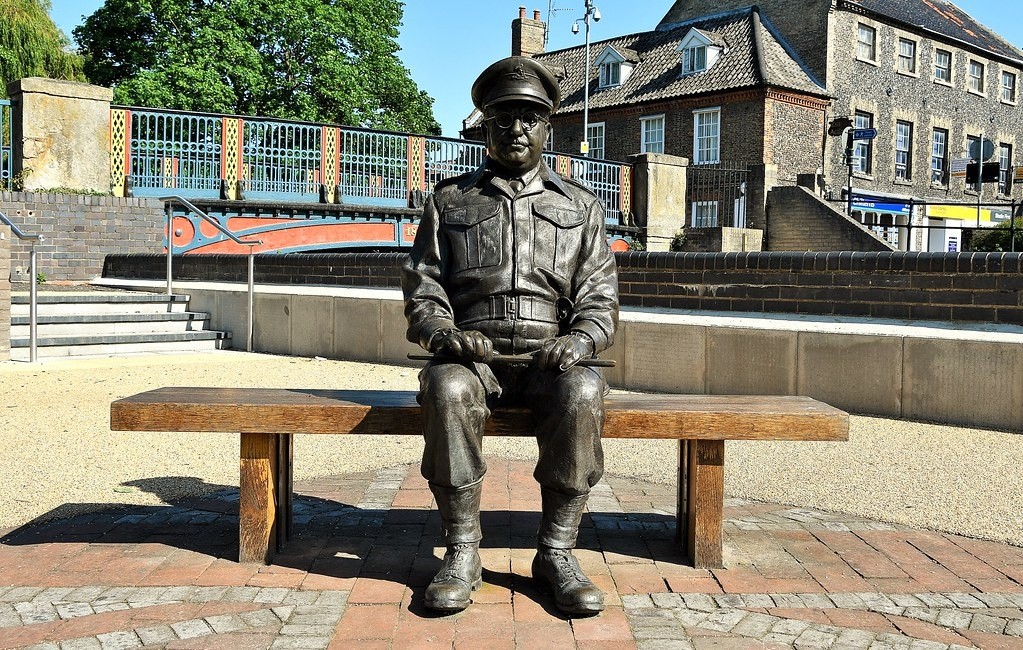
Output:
[[572, 0, 601, 157]]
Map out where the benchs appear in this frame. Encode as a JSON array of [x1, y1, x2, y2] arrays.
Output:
[[109, 386, 851, 567]]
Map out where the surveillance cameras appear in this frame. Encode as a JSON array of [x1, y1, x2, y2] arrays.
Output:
[[594, 11, 601, 22], [572, 24, 579, 34]]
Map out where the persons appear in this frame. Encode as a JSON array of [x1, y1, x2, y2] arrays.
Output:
[[402, 56, 620, 613]]
